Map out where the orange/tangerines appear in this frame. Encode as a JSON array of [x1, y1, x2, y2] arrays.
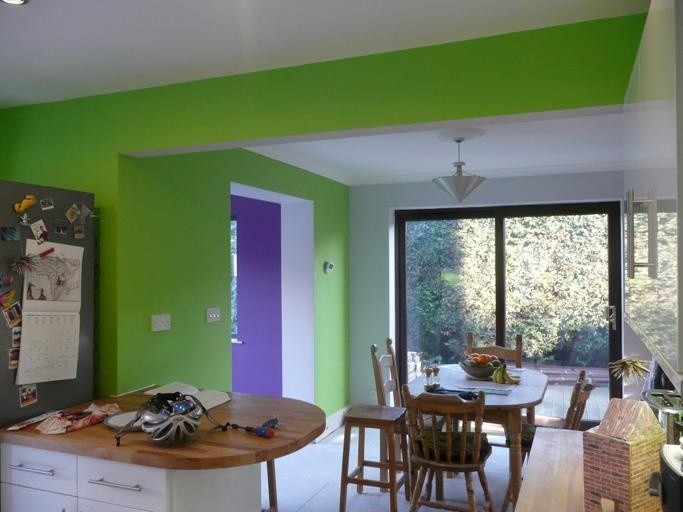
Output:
[[471, 352, 497, 363]]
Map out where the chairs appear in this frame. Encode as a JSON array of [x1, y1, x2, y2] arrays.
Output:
[[399, 383, 496, 512], [521, 367, 596, 430], [371, 336, 446, 492], [466, 329, 522, 448]]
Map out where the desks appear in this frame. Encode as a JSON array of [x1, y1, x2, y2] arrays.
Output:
[[0, 382, 328, 511], [514, 424, 585, 512]]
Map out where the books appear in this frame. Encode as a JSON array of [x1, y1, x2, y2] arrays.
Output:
[[143, 382, 231, 412]]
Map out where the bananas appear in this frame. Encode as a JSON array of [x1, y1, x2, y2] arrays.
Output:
[[491, 362, 521, 385]]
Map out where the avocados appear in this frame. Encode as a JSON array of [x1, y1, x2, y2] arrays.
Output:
[[462, 359, 501, 368]]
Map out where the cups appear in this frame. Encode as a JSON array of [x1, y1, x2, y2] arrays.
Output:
[[423, 359, 440, 390]]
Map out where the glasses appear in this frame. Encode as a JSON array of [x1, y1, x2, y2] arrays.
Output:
[[158, 400, 195, 417]]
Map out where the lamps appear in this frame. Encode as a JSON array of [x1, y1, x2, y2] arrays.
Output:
[[429, 136, 491, 203]]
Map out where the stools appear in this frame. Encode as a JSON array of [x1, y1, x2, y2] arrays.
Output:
[[339, 405, 409, 511]]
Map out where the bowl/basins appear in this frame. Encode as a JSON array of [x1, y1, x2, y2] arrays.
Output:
[[460, 363, 497, 378]]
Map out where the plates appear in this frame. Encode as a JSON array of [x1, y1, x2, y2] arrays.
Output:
[[102, 410, 141, 432]]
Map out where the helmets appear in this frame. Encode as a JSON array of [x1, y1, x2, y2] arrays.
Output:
[[137, 392, 205, 445]]
